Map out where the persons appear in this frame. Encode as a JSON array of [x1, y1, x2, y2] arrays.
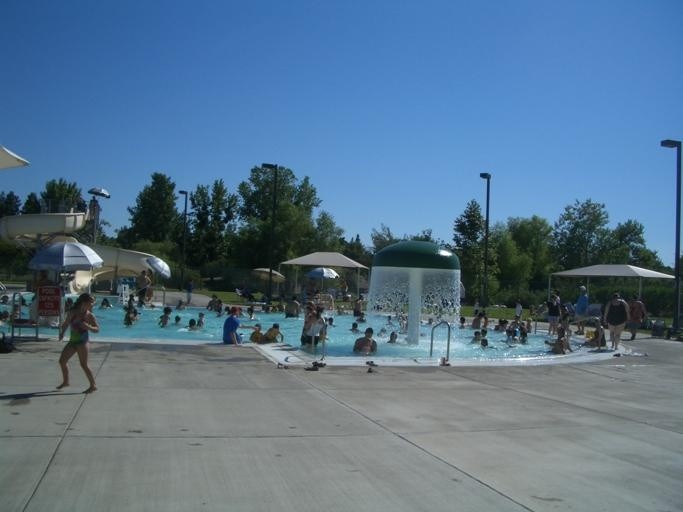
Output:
[[0, 271, 647, 355], [56, 293, 99, 393], [89, 196, 97, 208]]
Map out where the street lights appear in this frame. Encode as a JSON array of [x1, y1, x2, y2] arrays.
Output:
[[262, 163, 280, 305], [480, 172, 492, 309], [659, 139, 682, 329], [178, 189, 192, 293]]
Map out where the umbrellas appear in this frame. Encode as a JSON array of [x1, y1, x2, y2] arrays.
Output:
[[87, 186, 109, 202], [252, 266, 284, 281], [306, 267, 339, 288], [27, 241, 103, 292], [128, 256, 169, 289]]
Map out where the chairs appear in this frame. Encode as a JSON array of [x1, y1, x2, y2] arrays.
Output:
[[12, 285, 67, 342]]
